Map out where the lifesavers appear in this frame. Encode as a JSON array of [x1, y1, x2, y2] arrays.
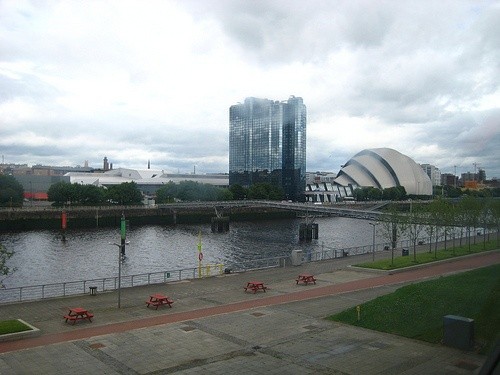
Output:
[[199, 252, 203, 260]]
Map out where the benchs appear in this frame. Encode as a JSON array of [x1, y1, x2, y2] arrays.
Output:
[[83, 312, 95, 323], [244, 287, 258, 293], [162, 299, 174, 308], [295, 279, 309, 286], [63, 314, 76, 325], [144, 300, 160, 310], [258, 285, 269, 293], [303, 278, 318, 285]]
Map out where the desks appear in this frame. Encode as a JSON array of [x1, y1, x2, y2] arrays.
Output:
[[296, 275, 316, 286], [147, 294, 172, 310], [66, 308, 92, 326], [245, 281, 267, 294]]
